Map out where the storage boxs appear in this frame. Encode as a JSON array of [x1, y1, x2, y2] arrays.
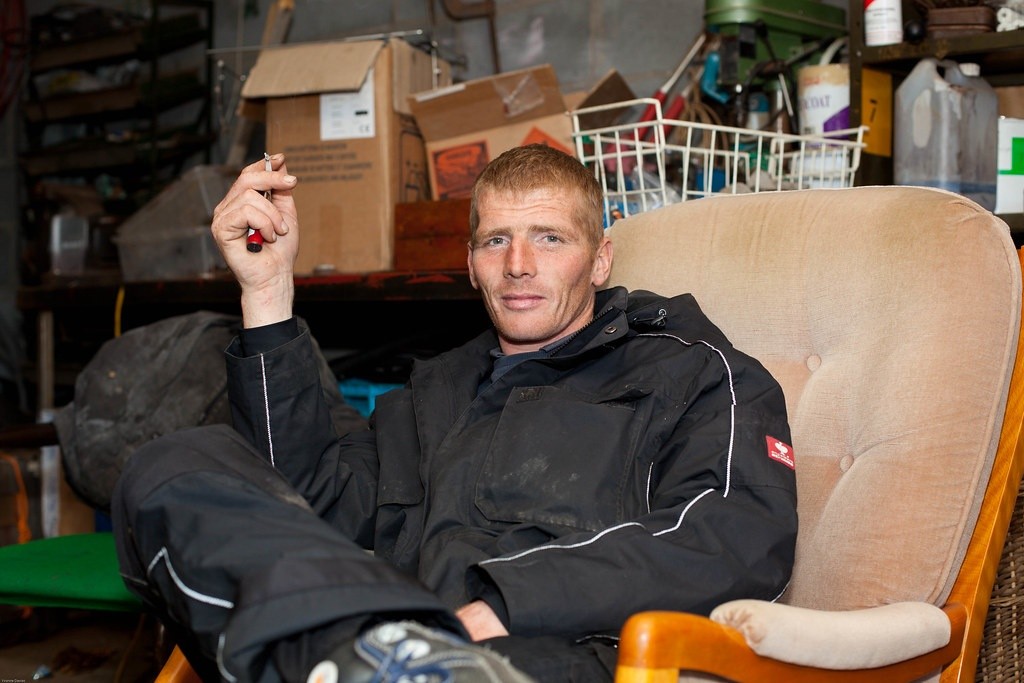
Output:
[[404, 64, 637, 202], [240, 38, 455, 279]]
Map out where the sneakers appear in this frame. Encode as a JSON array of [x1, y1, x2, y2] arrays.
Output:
[[303, 620, 536, 683]]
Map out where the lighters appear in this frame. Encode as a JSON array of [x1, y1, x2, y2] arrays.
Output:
[[246, 191, 272, 254]]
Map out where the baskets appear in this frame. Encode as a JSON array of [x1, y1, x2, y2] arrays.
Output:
[[564, 97, 868, 233]]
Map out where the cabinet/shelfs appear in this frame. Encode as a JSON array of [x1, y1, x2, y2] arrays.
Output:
[[847, 0, 1024, 228]]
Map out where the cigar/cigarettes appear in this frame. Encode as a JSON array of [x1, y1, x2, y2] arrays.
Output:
[[263, 152, 272, 172]]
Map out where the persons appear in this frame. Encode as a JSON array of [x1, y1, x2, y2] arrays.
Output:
[[111, 139, 801, 682]]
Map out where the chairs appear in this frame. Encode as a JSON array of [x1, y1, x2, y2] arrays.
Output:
[[0, 529, 197, 683], [605, 183, 1024, 683]]
